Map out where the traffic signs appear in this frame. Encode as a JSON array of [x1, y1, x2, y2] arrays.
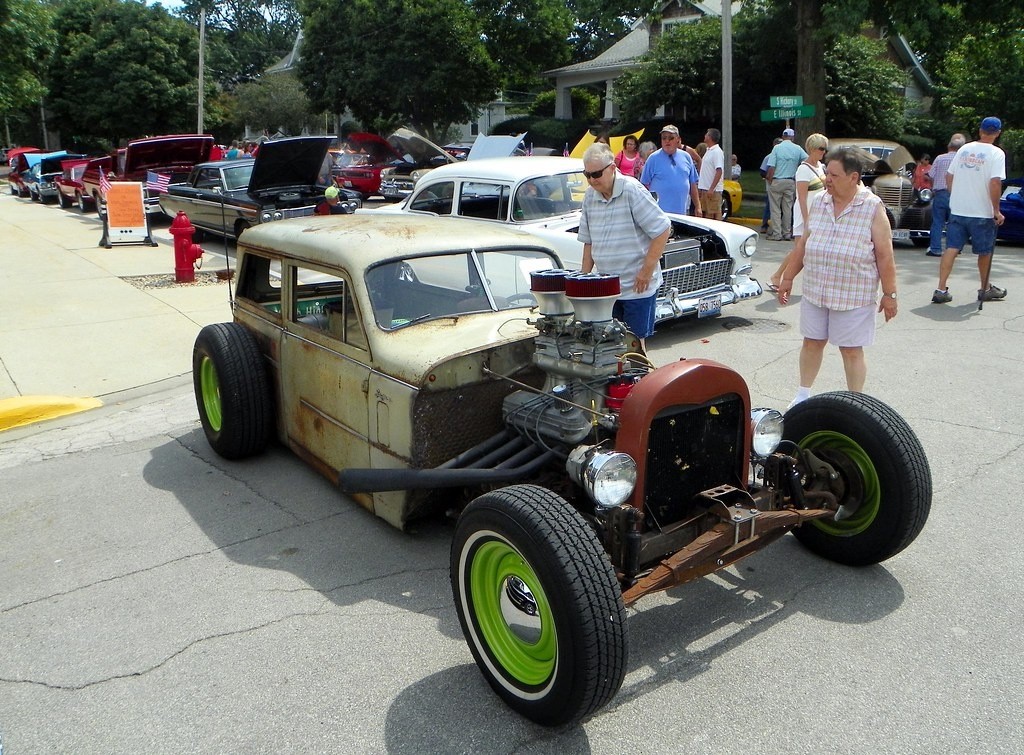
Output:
[[761, 104, 817, 122], [769, 95, 803, 108]]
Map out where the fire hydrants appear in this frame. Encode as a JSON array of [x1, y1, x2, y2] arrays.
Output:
[[166, 210, 205, 284]]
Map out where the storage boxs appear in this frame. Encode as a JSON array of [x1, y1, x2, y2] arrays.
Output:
[[324, 298, 394, 339]]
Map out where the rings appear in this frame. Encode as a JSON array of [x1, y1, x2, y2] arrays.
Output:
[[997, 223, 999, 224]]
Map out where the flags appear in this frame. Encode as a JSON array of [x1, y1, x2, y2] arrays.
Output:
[[99, 169, 112, 194], [563, 148, 569, 157], [146, 172, 171, 193]]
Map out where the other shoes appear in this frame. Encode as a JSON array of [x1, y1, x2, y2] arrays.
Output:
[[767, 236, 782, 240], [760, 229, 767, 233], [926, 251, 941, 257], [785, 235, 791, 240], [786, 396, 810, 411]]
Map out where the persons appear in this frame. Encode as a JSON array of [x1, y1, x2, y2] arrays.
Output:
[[931, 117, 1007, 302], [766, 133, 827, 292], [914, 134, 967, 256], [778, 147, 898, 410], [638, 125, 702, 218], [225, 139, 259, 159], [759, 138, 784, 233], [509, 132, 527, 156], [314, 186, 348, 215], [767, 128, 809, 240], [316, 152, 338, 185], [577, 142, 671, 352], [597, 129, 741, 221]]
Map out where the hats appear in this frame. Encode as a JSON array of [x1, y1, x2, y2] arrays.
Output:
[[981, 116, 1001, 132], [659, 125, 679, 138], [325, 186, 340, 198], [783, 129, 794, 136]]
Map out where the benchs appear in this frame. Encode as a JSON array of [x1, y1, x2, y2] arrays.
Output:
[[423, 197, 555, 219]]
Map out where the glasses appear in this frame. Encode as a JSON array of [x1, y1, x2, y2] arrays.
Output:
[[818, 147, 826, 151], [583, 163, 612, 179], [661, 136, 678, 140]]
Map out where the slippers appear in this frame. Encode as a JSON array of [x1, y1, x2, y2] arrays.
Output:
[[766, 282, 780, 292]]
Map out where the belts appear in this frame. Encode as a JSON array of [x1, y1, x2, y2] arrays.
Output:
[[772, 177, 795, 180]]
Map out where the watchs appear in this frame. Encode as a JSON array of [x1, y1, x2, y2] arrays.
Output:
[[884, 292, 896, 299]]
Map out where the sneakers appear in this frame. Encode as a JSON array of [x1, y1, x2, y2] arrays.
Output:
[[932, 287, 952, 303], [978, 284, 1007, 300]]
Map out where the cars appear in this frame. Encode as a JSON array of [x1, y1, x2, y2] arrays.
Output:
[[315, 128, 560, 205], [157, 134, 363, 243], [353, 156, 764, 330], [7, 133, 223, 223], [547, 127, 744, 220], [819, 138, 949, 248], [191, 214, 937, 729]]
